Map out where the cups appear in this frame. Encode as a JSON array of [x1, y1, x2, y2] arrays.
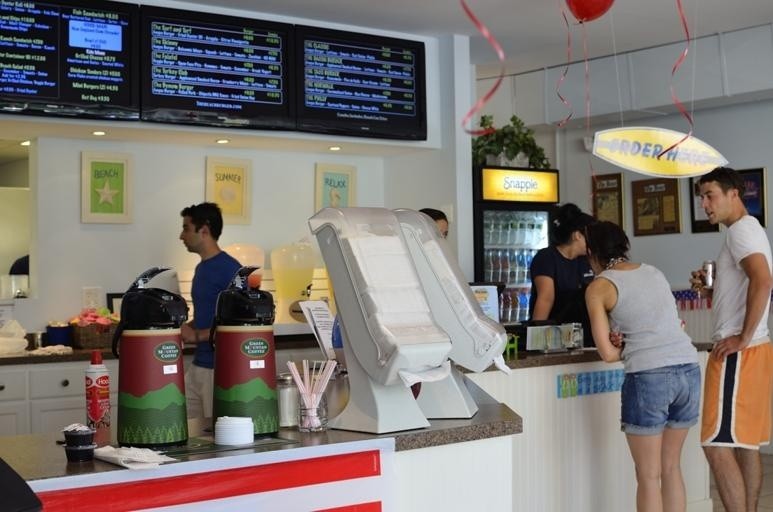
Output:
[[24, 333, 47, 350], [45, 326, 70, 347], [298, 391, 329, 434], [213, 416, 254, 445], [62, 429, 97, 464]]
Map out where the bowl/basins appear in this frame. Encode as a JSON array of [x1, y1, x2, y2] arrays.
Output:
[[69, 322, 119, 350]]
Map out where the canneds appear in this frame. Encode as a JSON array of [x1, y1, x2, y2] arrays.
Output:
[[702, 261, 714, 288]]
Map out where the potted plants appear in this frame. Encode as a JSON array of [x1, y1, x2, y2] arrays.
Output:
[[473, 116, 550, 169]]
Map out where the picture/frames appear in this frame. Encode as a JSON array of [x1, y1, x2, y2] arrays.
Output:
[[631, 177, 683, 237], [81, 152, 135, 224], [689, 166, 768, 233], [314, 165, 354, 214], [591, 171, 625, 234], [204, 155, 253, 225]]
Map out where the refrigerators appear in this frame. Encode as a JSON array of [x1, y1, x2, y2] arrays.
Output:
[[472, 166, 560, 353]]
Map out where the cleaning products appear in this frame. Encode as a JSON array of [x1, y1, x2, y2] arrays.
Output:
[[84, 349, 112, 431]]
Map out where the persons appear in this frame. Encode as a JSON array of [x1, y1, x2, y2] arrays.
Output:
[[176, 202, 246, 419], [526, 202, 597, 348], [689, 167, 773, 512], [584, 221, 701, 511], [333, 207, 451, 375]]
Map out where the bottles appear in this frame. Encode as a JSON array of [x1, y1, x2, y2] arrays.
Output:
[[278, 372, 298, 428], [84, 350, 112, 447], [480, 210, 546, 323]]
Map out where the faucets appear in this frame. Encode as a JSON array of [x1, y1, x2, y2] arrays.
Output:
[[301, 283, 313, 297]]
